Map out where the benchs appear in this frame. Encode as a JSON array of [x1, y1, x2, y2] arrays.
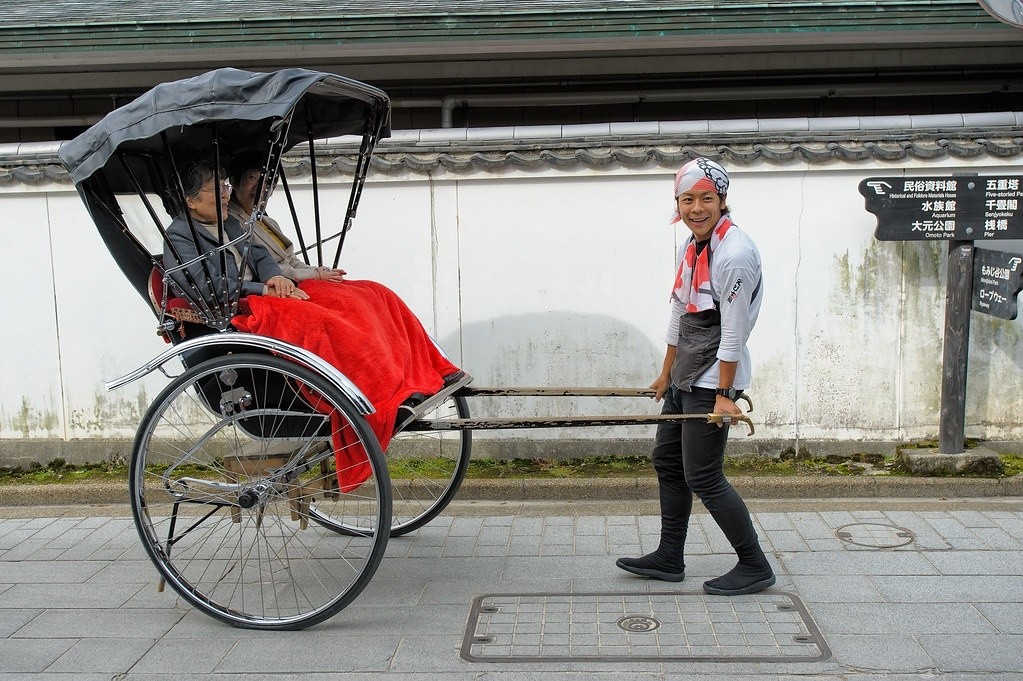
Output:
[[147, 265, 313, 421]]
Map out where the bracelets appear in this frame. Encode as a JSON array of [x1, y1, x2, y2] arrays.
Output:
[[315, 268, 321, 280]]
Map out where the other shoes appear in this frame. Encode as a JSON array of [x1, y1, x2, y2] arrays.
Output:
[[443, 370, 465, 387], [405, 392, 429, 407]]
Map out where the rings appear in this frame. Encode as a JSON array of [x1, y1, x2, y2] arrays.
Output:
[[291, 291, 295, 294]]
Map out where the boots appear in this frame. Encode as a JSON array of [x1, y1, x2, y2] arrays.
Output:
[[615, 532, 687, 582], [702, 541, 776, 596]]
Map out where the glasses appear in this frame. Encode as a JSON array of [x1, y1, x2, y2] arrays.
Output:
[[205, 183, 233, 195]]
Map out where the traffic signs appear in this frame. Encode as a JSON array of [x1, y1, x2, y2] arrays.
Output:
[[968, 247, 1023, 322], [857, 173, 1023, 241]]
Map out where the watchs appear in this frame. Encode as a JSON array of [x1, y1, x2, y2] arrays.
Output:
[[716, 387, 735, 400]]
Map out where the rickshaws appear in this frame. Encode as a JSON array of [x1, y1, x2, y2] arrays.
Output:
[[57, 66, 764, 633]]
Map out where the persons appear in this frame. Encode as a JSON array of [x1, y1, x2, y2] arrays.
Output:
[[163, 152, 434, 425], [616, 157, 775, 594], [224, 152, 465, 394]]
